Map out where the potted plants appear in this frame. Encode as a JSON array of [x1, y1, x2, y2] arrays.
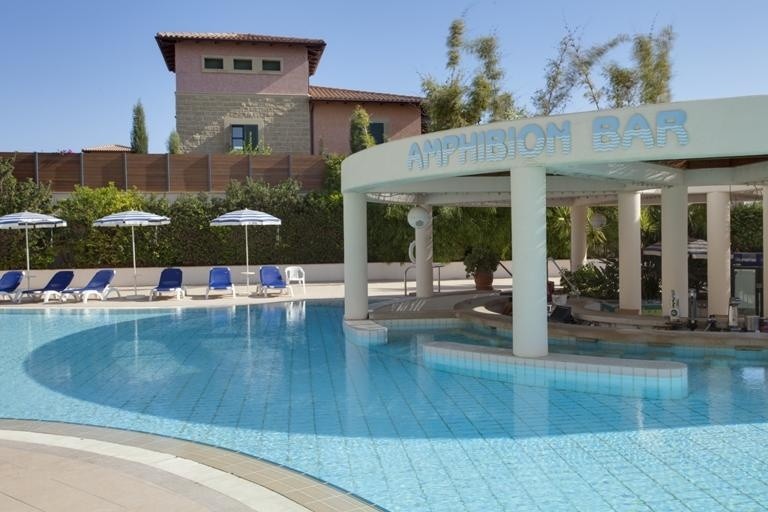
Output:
[[464, 247, 500, 290]]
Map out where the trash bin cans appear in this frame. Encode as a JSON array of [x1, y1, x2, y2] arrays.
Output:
[[728, 296, 742, 328], [688, 288, 697, 318]]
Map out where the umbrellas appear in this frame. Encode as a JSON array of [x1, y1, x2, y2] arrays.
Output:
[[0, 210, 68, 297], [643, 235, 707, 259], [209, 208, 282, 289], [91, 209, 171, 295]]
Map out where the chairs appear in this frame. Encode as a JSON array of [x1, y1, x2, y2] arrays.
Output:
[[0, 269, 121, 304], [257, 265, 294, 297], [149, 269, 187, 301], [285, 267, 305, 293], [205, 267, 237, 300]]
